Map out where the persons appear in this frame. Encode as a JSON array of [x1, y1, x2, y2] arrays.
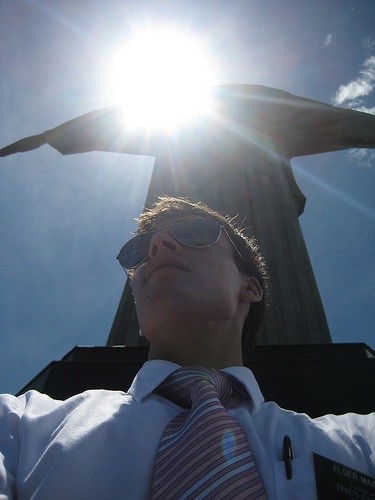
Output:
[[0, 83, 375, 346], [0, 196, 375, 500]]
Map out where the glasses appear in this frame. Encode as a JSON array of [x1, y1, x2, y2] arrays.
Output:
[[115, 215, 253, 283]]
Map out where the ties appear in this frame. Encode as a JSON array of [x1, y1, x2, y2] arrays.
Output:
[[151, 364, 269, 500]]
